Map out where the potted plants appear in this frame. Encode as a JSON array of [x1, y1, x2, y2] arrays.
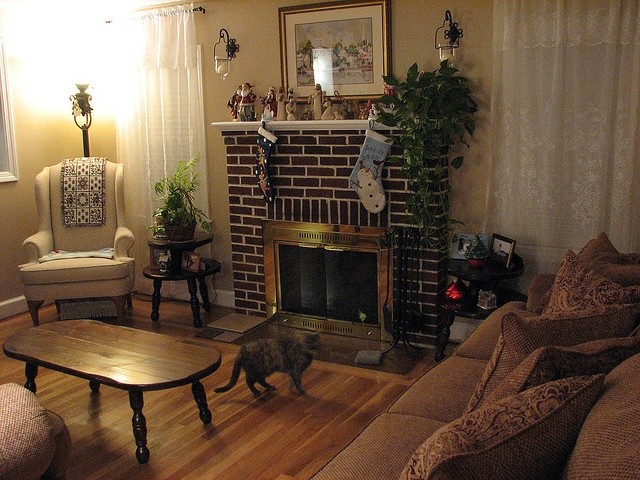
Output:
[[144, 151, 213, 243], [368, 57, 479, 250]]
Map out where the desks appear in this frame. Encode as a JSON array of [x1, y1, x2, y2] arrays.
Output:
[[141, 232, 223, 328]]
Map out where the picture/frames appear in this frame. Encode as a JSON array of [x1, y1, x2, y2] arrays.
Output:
[[145, 244, 170, 269], [180, 249, 200, 275], [485, 233, 516, 270], [450, 232, 488, 260], [277, 1, 393, 103]]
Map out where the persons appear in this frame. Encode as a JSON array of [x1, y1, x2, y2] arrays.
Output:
[[240, 83, 256, 121]]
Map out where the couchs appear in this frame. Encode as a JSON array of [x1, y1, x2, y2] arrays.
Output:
[[308, 269, 639, 479], [0, 381, 72, 479], [17, 157, 136, 326]]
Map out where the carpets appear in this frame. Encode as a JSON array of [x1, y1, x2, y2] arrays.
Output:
[[195, 311, 428, 377]]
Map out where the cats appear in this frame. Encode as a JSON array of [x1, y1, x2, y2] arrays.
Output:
[[213, 334, 325, 399]]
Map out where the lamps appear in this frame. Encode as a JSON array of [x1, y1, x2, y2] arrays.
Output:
[[70, 82, 92, 156], [432, 9, 463, 68], [211, 26, 239, 84]]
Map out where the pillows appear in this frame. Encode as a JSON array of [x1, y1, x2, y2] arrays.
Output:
[[594, 230, 622, 254], [397, 371, 606, 476], [540, 248, 640, 315], [479, 337, 640, 409], [459, 299, 639, 415], [527, 238, 640, 317]]
